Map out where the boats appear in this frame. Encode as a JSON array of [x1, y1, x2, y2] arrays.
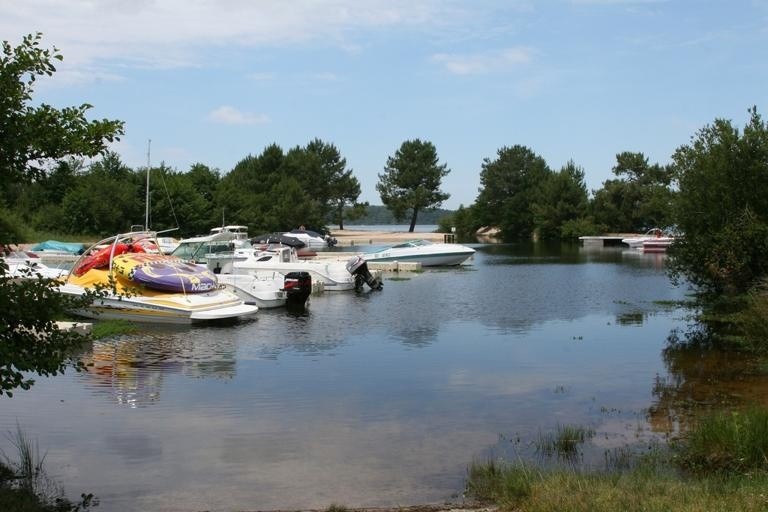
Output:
[[622, 228, 688, 248], [0, 140, 384, 326], [344, 236, 476, 267]]
